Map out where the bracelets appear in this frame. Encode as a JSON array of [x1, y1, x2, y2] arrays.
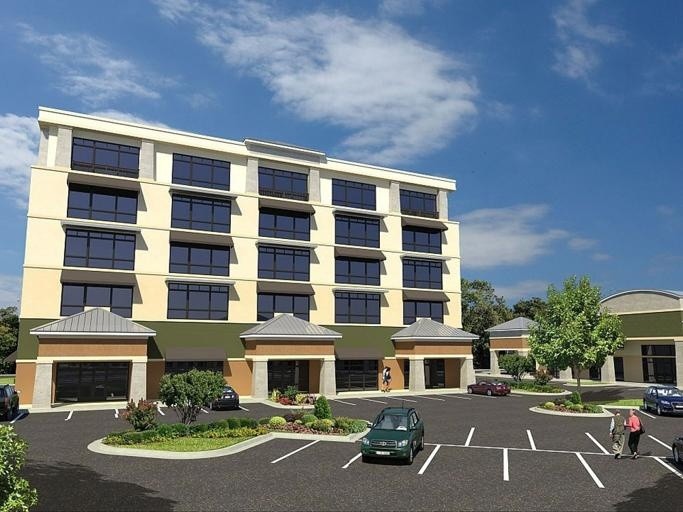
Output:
[[609, 433, 612, 434]]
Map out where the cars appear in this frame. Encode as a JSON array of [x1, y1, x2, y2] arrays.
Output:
[[671, 433, 683, 466], [466, 379, 511, 397], [357, 405, 426, 466], [204, 385, 240, 410]]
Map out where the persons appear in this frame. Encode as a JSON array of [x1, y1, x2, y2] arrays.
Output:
[[381, 366, 388, 391], [625, 407, 642, 460], [384, 366, 392, 392], [607, 408, 627, 459]]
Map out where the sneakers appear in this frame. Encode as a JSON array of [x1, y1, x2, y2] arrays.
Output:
[[630, 454, 637, 459], [614, 452, 620, 459], [637, 452, 640, 458]]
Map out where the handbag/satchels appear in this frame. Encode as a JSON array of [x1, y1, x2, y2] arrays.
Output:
[[637, 417, 645, 435]]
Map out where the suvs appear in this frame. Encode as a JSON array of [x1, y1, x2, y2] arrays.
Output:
[[0, 383, 21, 422], [642, 384, 683, 416]]
